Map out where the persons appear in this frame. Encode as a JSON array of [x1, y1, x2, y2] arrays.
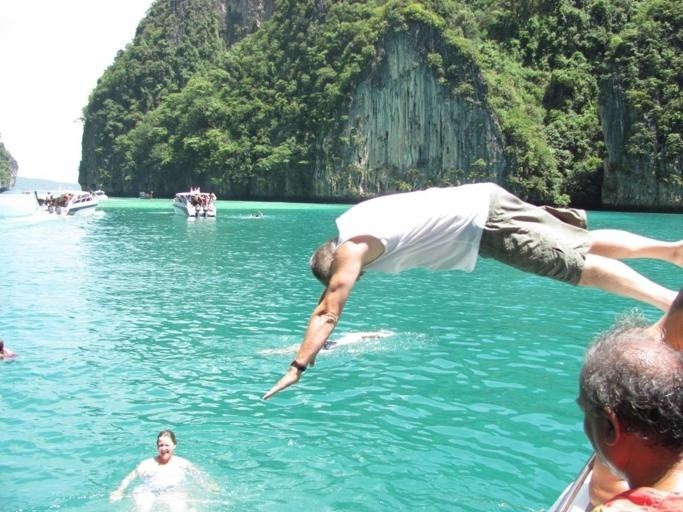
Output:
[[0, 341, 15, 360], [190, 190, 217, 218], [262, 181, 683, 400], [109, 430, 219, 512], [45, 192, 72, 214], [257, 328, 407, 358], [586, 289, 683, 512], [576, 306, 683, 512]]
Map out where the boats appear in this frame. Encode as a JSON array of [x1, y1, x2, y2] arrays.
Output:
[[139, 191, 153, 198], [0, 190, 108, 218], [173, 187, 217, 217]]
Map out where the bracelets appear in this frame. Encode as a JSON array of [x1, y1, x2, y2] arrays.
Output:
[[289, 361, 306, 372]]
